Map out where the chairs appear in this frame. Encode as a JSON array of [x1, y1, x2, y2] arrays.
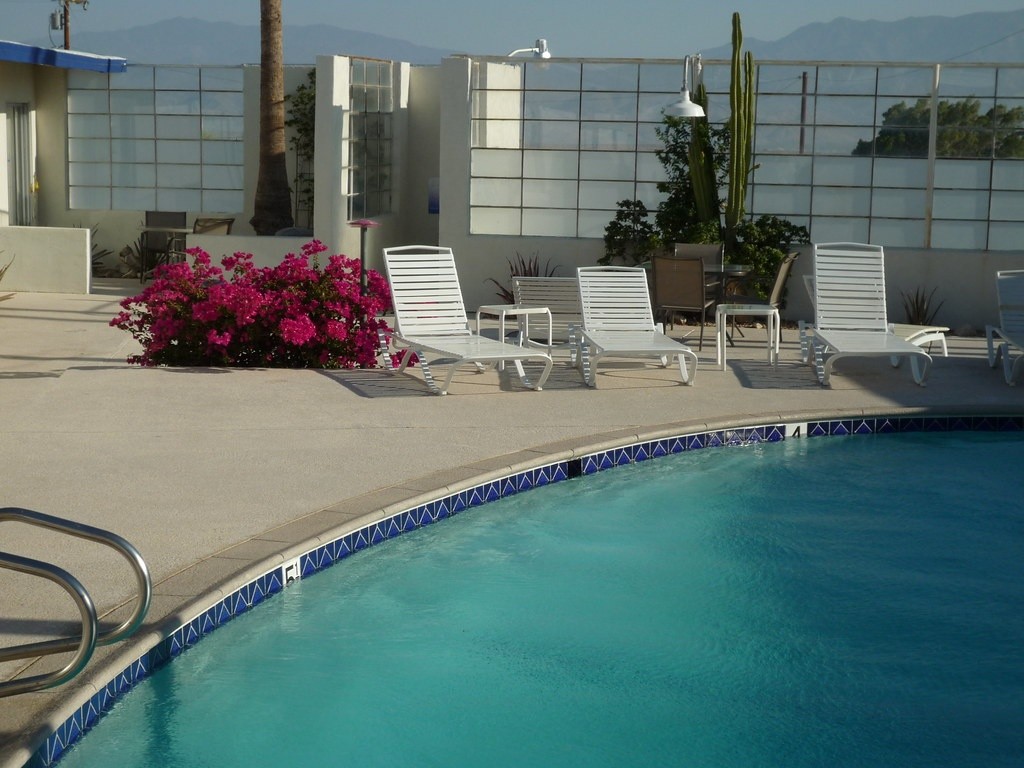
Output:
[[167, 215, 235, 266], [378, 240, 1024, 396], [140, 211, 186, 284]]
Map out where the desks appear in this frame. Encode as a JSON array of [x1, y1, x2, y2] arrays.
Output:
[[476, 304, 553, 372], [138, 226, 194, 284], [717, 303, 781, 372]]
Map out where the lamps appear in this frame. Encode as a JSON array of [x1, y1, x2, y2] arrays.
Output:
[[663, 53, 708, 117], [501, 38, 550, 70]]
